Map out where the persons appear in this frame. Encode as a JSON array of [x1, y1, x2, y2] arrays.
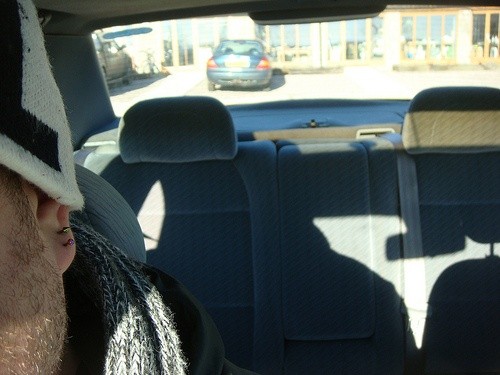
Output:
[[0, 0, 258, 375]]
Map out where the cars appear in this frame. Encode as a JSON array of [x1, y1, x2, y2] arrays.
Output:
[[206, 40, 273, 92], [97, 39, 132, 86]]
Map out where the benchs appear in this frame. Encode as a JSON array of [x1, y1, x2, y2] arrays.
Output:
[[74, 87, 500, 375]]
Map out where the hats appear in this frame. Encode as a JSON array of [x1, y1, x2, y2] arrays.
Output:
[[0, 0, 84, 210]]
